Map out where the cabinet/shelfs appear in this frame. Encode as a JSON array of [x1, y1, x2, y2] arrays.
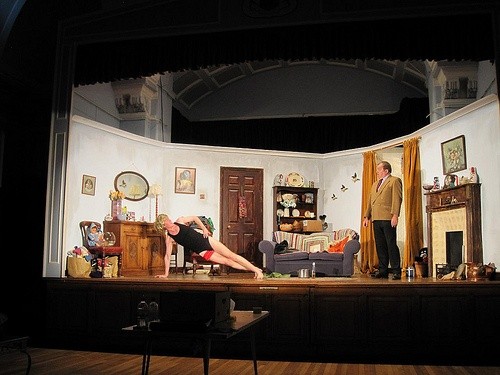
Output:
[[272, 186, 320, 234], [104, 220, 165, 277]]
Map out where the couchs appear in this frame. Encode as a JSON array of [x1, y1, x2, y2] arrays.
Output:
[[258, 229, 360, 277]]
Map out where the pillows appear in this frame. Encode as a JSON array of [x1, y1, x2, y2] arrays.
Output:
[[328, 236, 349, 252]]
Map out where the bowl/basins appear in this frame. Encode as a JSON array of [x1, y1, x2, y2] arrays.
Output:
[[299, 268, 310, 278]]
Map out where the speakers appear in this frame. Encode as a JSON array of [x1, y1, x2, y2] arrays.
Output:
[[158, 290, 230, 325]]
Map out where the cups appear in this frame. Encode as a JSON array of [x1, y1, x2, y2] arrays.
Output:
[[309, 181, 314, 188], [117, 214, 125, 220]]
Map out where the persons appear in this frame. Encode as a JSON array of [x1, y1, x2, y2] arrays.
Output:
[[86, 179, 93, 189], [178, 170, 192, 189], [362, 161, 403, 280], [153, 214, 263, 280]]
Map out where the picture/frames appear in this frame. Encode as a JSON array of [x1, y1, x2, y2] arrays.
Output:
[[82, 175, 96, 196], [174, 167, 196, 194], [441, 135, 467, 175]]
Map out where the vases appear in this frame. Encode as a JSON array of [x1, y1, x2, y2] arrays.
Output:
[[111, 201, 122, 220]]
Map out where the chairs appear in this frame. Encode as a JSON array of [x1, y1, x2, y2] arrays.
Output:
[[169, 241, 178, 274], [182, 215, 222, 276], [80, 221, 122, 278]]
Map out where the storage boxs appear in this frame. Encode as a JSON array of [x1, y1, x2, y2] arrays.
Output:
[[302, 220, 323, 232]]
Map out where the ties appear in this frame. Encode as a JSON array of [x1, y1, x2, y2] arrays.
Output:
[[375, 179, 383, 192]]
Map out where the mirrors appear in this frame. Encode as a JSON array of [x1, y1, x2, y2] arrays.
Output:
[[114, 171, 149, 202]]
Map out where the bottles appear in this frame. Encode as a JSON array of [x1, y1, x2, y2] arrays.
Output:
[[279, 174, 284, 186], [312, 262, 316, 279], [468, 167, 477, 183], [274, 175, 280, 186], [433, 177, 440, 191]]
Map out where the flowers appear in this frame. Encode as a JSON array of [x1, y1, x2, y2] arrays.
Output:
[[108, 190, 125, 200]]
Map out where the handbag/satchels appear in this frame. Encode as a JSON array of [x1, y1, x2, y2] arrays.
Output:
[[67, 251, 92, 277]]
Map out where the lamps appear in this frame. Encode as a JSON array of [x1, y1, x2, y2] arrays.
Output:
[[147, 184, 165, 220], [128, 184, 142, 199]]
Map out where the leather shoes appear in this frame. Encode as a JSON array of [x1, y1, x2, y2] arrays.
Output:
[[371, 271, 389, 278], [392, 273, 401, 280]]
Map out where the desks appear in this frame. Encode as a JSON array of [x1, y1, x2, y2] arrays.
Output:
[[122, 309, 271, 375], [0, 336, 32, 375]]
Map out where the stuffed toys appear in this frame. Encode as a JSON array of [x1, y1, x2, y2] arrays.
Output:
[[88, 223, 99, 246]]
